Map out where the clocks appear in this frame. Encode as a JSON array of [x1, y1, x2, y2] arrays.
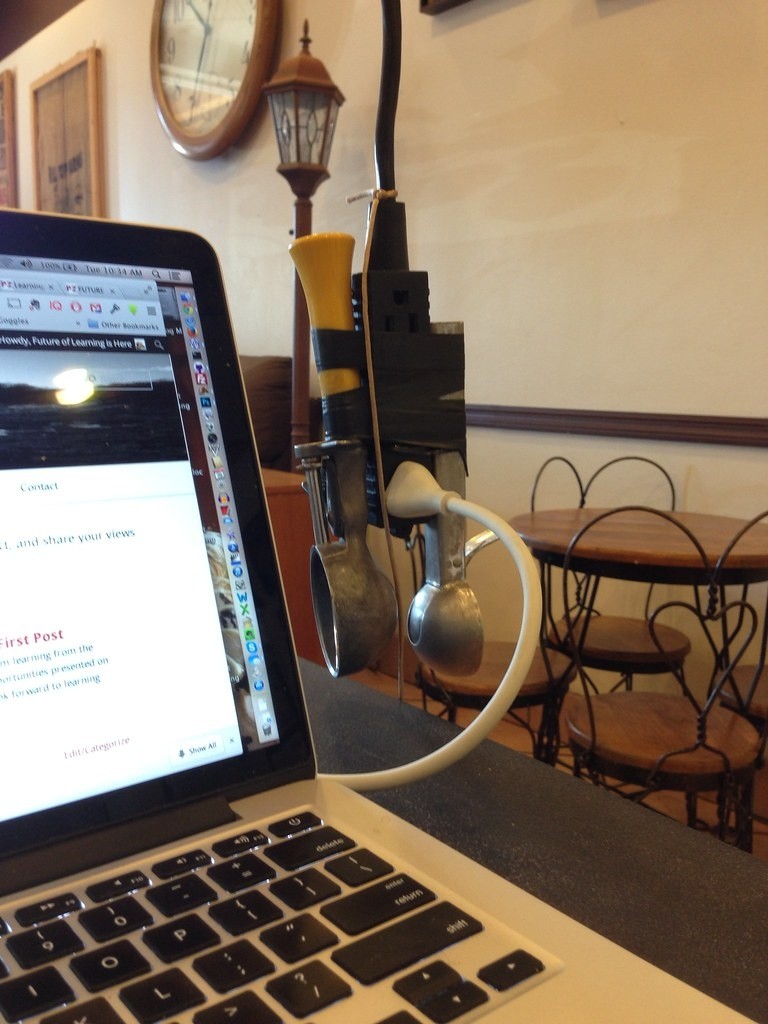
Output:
[[149, 1, 279, 162]]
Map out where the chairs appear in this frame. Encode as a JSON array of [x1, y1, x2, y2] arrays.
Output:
[[405, 509, 579, 770], [530, 457, 691, 780], [712, 665, 767, 856], [563, 506, 768, 853]]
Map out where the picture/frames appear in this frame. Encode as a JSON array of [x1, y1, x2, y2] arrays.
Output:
[[30, 47, 106, 218], [0, 69, 18, 209]]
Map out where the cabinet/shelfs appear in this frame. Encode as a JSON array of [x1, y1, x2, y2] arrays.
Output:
[[259, 464, 327, 669]]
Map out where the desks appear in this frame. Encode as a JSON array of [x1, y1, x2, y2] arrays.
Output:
[[286, 652, 768, 1024], [507, 506, 768, 771]]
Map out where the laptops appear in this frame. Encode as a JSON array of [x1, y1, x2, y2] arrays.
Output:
[[0, 207, 758, 1024]]
